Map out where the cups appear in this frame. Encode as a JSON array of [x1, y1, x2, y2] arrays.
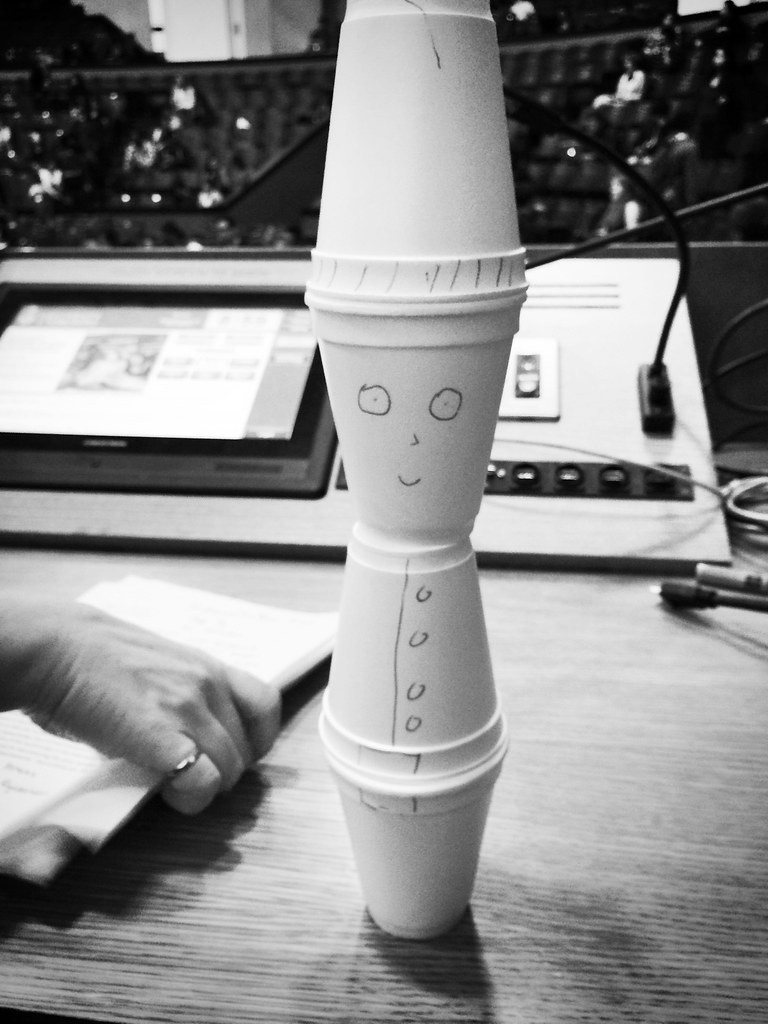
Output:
[[321, 741, 508, 940], [305, 0, 526, 304], [304, 294, 529, 542], [318, 527, 509, 785]]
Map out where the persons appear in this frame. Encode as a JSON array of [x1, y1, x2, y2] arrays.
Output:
[[1, 588, 284, 819], [0, 3, 766, 250]]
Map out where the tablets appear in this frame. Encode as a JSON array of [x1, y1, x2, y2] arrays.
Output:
[[0, 282, 337, 502]]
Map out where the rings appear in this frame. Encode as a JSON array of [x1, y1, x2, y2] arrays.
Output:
[[165, 746, 201, 779]]
